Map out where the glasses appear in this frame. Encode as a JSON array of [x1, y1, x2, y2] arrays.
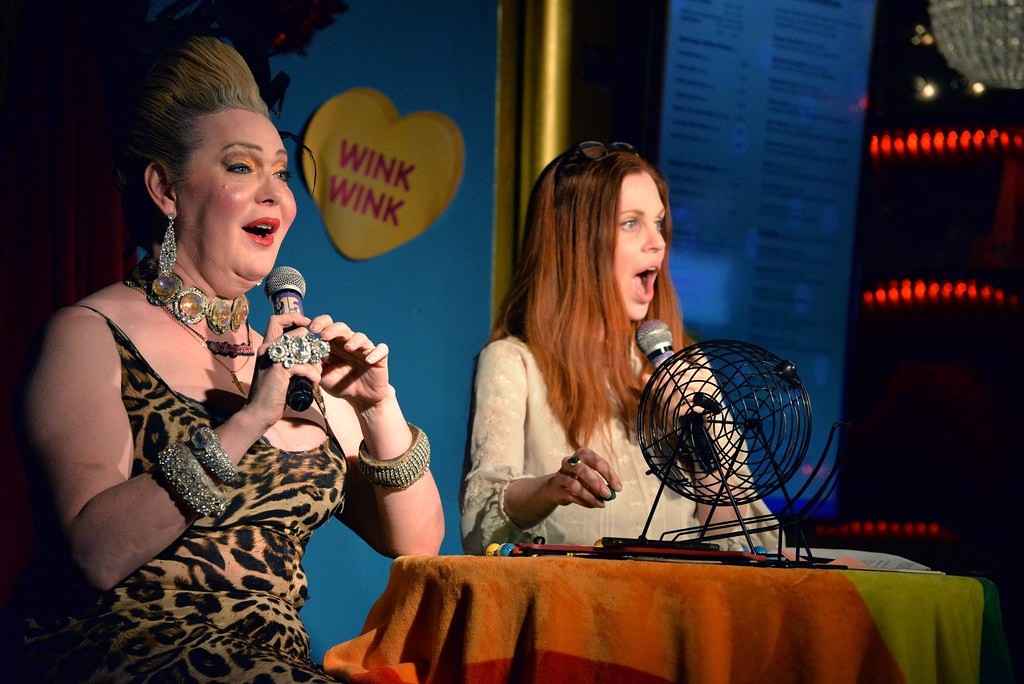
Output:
[[556, 139, 642, 175]]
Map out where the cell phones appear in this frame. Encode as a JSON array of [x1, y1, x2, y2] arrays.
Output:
[[600, 537, 720, 552]]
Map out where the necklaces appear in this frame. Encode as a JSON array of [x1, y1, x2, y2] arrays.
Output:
[[134, 254, 255, 397]]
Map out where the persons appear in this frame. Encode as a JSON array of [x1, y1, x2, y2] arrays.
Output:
[[16, 33, 449, 684], [456, 141, 787, 560]]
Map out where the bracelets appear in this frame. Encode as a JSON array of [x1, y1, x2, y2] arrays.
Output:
[[191, 425, 240, 485], [357, 420, 431, 491], [155, 439, 228, 518]]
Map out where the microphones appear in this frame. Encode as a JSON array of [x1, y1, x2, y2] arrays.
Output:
[[264, 265, 315, 412], [635, 317, 719, 475]]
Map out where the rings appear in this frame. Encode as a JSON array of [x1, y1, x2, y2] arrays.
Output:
[[267, 333, 331, 369], [568, 455, 583, 466]]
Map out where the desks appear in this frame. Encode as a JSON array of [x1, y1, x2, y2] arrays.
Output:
[[391, 552, 1006, 684]]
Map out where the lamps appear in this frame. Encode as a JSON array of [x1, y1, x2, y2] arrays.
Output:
[[929, 1, 1023, 91]]
